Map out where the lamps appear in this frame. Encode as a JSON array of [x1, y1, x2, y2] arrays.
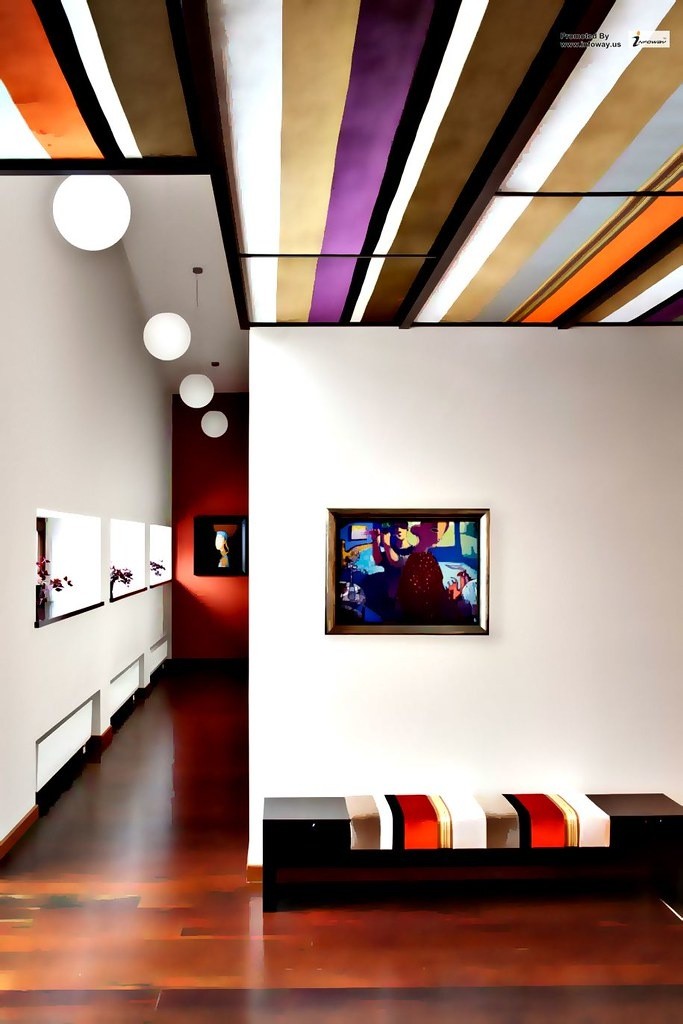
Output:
[[201, 411, 228, 438], [179, 374, 214, 408], [52, 175, 131, 251], [142, 312, 192, 361]]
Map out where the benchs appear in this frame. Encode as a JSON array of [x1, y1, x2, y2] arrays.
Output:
[[263, 793, 683, 911]]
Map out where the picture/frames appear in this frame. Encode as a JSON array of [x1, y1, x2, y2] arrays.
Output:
[[324, 507, 490, 637], [193, 514, 248, 576]]
